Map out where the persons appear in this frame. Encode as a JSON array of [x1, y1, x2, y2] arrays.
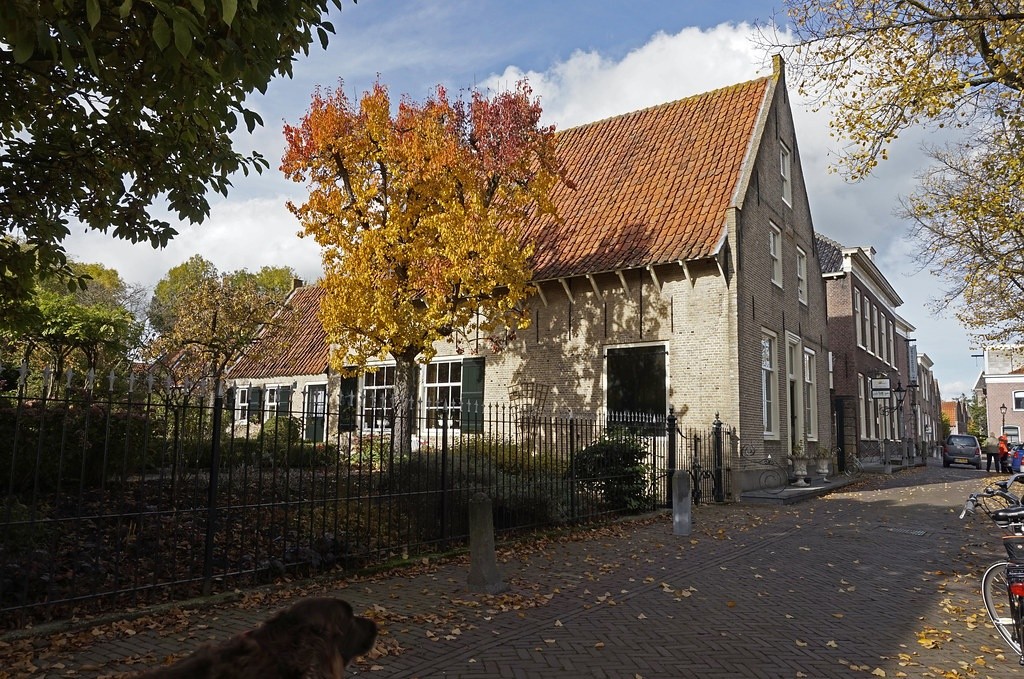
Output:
[[985, 432, 1000, 473], [997, 435, 1015, 474]]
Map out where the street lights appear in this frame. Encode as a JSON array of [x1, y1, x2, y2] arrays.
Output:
[[1000, 403, 1007, 434]]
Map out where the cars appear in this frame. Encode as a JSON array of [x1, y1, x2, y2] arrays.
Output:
[[1007, 443, 1024, 473], [943, 434, 982, 470]]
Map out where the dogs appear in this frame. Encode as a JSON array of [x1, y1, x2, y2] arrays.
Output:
[[130, 596, 380, 678]]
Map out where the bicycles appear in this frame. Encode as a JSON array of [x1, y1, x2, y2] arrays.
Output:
[[959, 472, 1024, 666]]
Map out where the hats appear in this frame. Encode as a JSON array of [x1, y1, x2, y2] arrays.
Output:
[[990, 432, 995, 436]]
